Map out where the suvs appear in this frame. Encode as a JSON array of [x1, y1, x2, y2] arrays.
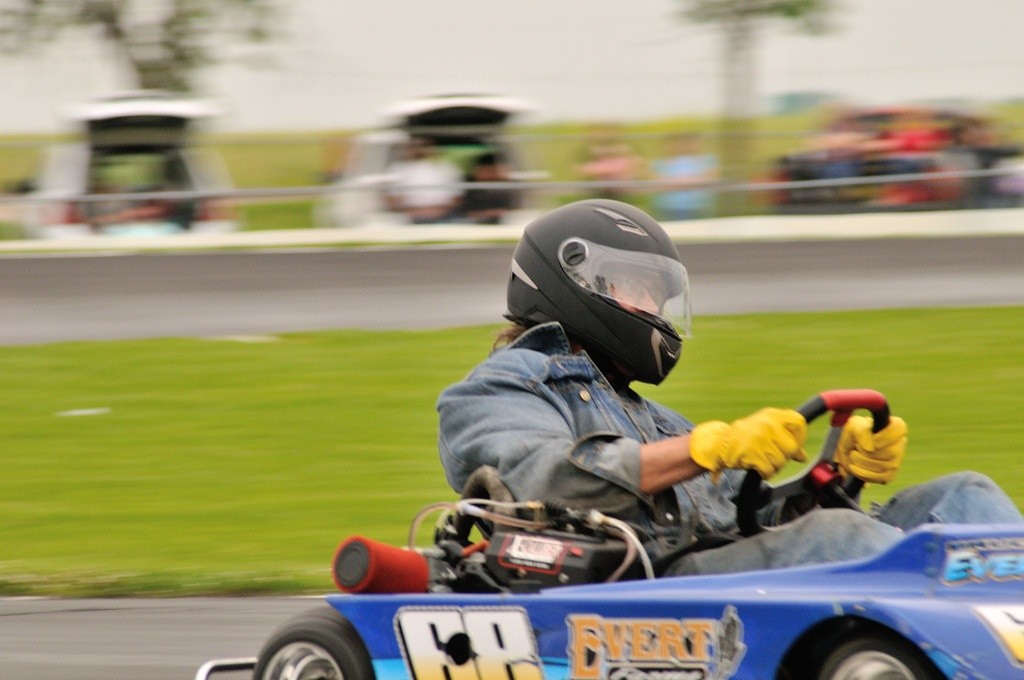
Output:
[[320, 95, 545, 232], [12, 94, 244, 236]]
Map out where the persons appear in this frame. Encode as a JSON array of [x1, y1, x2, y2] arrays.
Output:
[[431, 213, 906, 554], [648, 132, 722, 219], [574, 124, 648, 200]]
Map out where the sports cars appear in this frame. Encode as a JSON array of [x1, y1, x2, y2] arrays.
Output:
[[769, 110, 1007, 207]]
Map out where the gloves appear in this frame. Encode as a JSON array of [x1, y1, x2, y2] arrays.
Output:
[[691, 406, 806, 478], [835, 412, 908, 482]]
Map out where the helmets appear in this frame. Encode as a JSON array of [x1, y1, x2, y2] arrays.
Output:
[[502, 200, 691, 378]]
